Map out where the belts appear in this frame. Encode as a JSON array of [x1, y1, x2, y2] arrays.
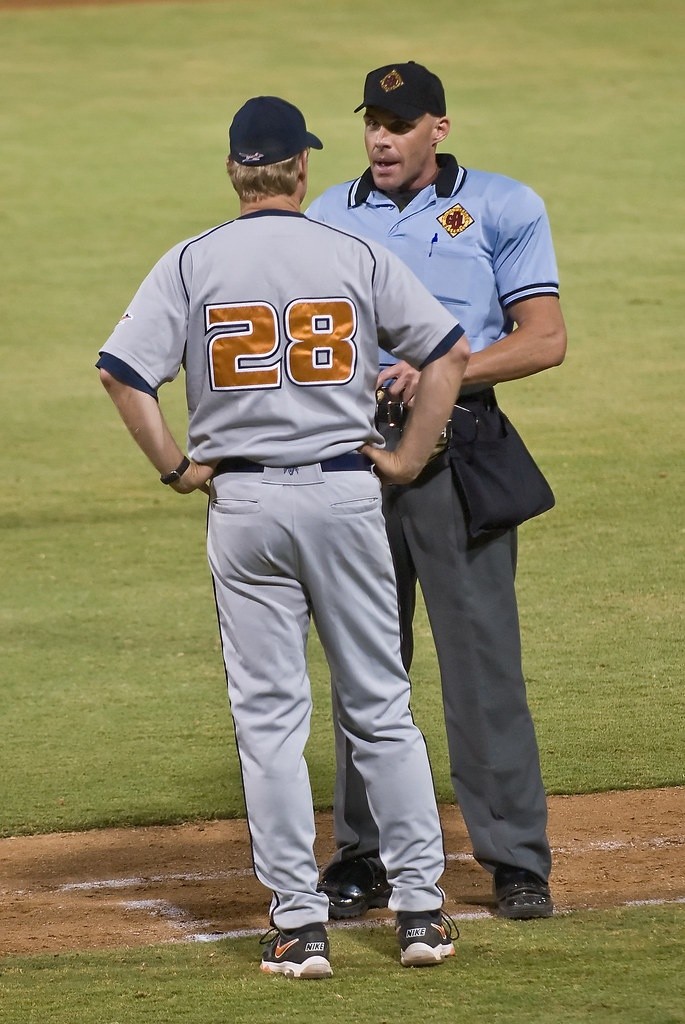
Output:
[[374, 400, 407, 426], [206, 453, 375, 478]]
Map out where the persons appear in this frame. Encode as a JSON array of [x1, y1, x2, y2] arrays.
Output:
[[304, 59, 569, 919], [96, 97, 470, 976]]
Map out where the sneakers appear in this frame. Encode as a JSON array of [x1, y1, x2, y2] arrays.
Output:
[[259, 923, 334, 979], [396, 907, 459, 965]]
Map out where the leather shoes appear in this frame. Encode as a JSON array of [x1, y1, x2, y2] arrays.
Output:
[[317, 858, 392, 919], [493, 864, 552, 917]]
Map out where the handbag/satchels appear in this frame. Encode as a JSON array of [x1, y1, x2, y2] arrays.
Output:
[[453, 412, 555, 550]]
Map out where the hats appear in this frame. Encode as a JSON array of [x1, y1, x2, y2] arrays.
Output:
[[229, 96, 323, 166], [354, 61, 443, 120]]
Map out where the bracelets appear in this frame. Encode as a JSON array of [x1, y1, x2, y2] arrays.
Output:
[[160, 455, 190, 485]]
